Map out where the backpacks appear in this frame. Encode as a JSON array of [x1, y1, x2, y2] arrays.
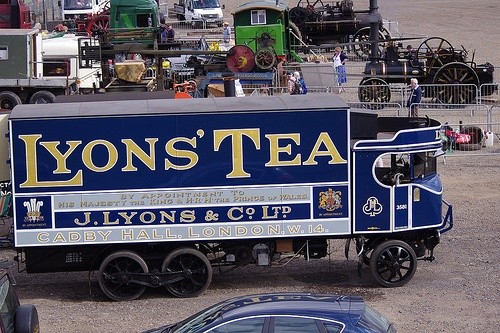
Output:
[[289, 77, 302, 95]]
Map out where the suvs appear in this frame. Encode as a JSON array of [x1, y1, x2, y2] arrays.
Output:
[[144, 288, 398, 333]]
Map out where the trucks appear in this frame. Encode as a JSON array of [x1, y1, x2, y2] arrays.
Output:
[[173, 0, 226, 28], [7, 91, 455, 302]]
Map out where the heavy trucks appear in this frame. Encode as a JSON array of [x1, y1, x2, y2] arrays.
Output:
[[57, 0, 110, 30], [0, 0, 33, 31], [105, 0, 161, 49], [0, 26, 103, 112]]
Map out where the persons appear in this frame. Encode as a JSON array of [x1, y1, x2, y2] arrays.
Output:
[[406, 78, 422, 117], [332, 47, 348, 93], [294, 72, 307, 94], [167, 26, 174, 43], [287, 72, 297, 95], [224, 22, 231, 43]]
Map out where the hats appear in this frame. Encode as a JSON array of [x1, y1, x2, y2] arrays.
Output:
[[286, 70, 292, 75]]
